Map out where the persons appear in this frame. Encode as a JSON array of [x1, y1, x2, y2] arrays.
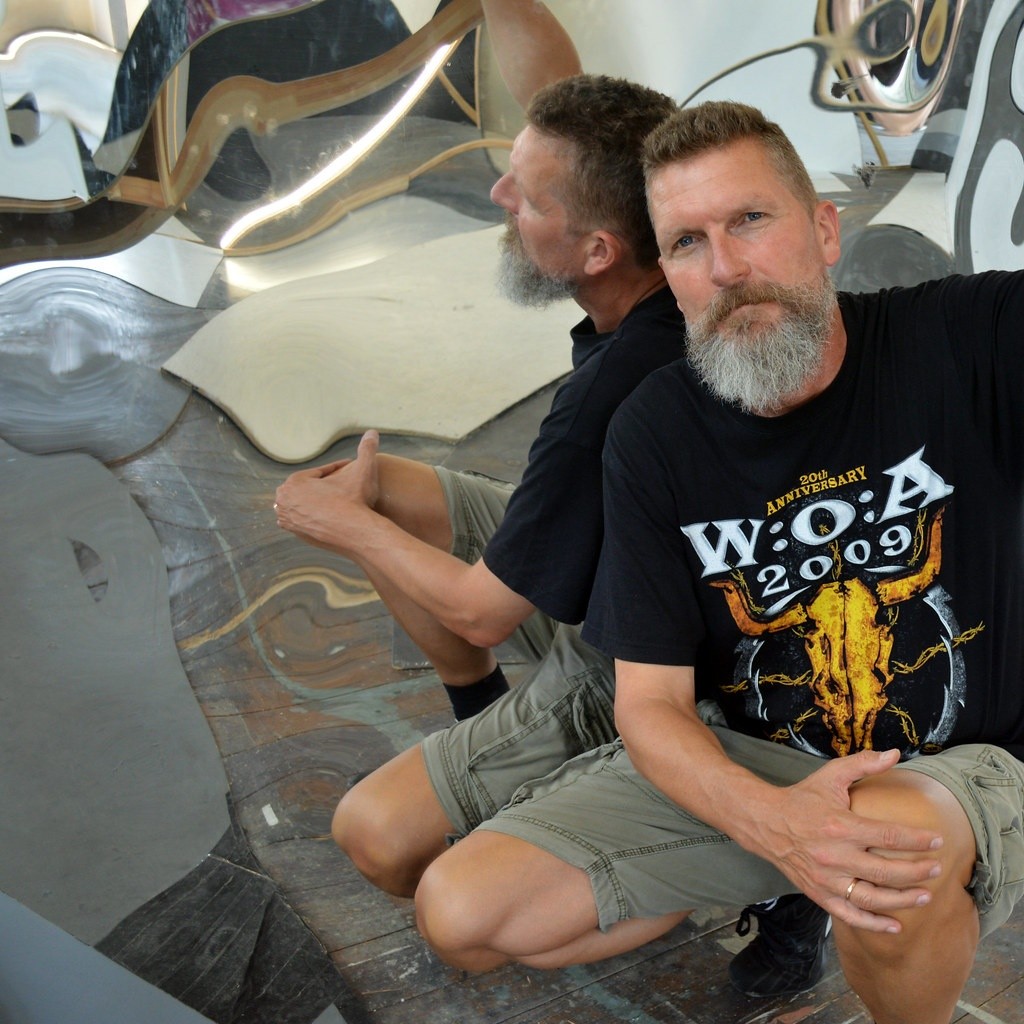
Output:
[[267, 75, 689, 894], [411, 100, 1023, 1024]]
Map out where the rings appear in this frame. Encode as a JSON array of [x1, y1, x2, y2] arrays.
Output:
[[843, 877, 862, 902], [274, 503, 277, 508]]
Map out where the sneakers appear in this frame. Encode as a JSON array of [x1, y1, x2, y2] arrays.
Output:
[[728, 893, 833, 999]]
